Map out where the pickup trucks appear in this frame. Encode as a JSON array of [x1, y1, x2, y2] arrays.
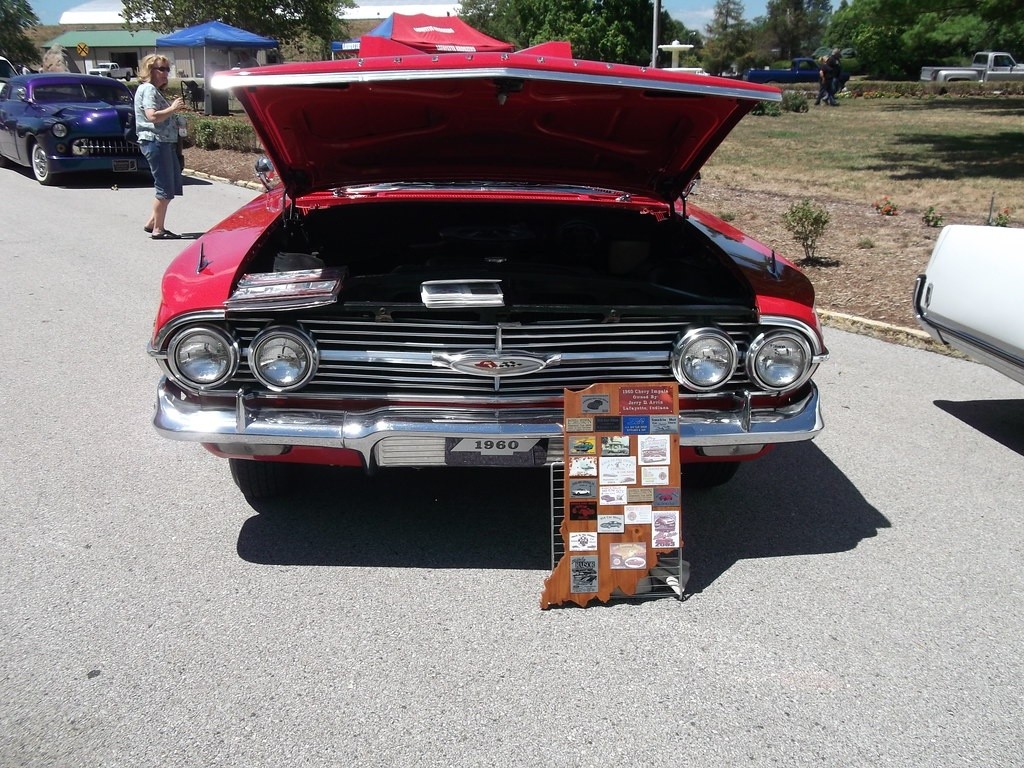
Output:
[[742, 57, 822, 85], [89, 62, 134, 82], [920, 52, 1024, 85]]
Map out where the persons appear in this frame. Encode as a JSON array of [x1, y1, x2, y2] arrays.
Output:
[[235, 50, 260, 68], [16, 63, 44, 74], [816, 49, 845, 108], [133, 54, 188, 241]]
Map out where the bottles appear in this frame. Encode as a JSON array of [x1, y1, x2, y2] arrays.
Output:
[[178, 113, 188, 137]]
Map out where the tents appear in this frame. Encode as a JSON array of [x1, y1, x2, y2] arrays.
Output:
[[154, 20, 279, 114], [331, 13, 514, 62]]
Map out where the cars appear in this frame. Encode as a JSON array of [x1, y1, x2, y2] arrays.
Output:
[[0, 54, 33, 93], [0, 72, 185, 187], [912, 223, 1023, 382]]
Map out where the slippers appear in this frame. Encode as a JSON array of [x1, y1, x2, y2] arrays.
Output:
[[144, 225, 153, 233], [151, 230, 181, 239]]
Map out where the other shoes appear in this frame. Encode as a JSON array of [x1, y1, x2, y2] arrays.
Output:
[[830, 101, 840, 105], [814, 101, 820, 105], [822, 97, 828, 104]]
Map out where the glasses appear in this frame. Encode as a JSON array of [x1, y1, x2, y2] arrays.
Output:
[[152, 67, 170, 72]]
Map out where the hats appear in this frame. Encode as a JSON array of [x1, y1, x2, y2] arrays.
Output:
[[822, 55, 831, 62], [831, 48, 840, 56]]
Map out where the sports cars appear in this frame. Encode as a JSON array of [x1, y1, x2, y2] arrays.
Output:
[[147, 41, 832, 502]]
